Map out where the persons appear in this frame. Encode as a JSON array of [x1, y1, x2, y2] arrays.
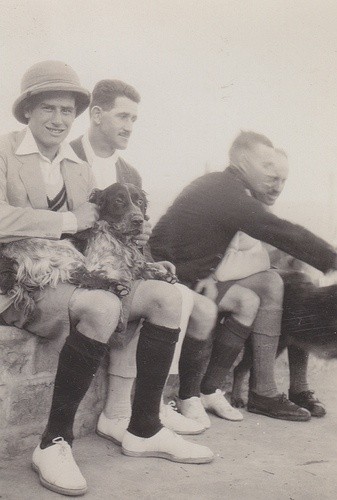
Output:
[[0, 61, 212, 497], [70, 80, 206, 446], [214, 146, 326, 421], [143, 131, 337, 423]]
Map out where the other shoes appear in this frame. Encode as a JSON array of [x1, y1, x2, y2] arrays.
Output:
[[177, 396, 211, 428], [31, 437, 87, 496], [122, 426, 214, 463], [159, 400, 205, 434], [96, 412, 131, 447], [200, 389, 244, 420]]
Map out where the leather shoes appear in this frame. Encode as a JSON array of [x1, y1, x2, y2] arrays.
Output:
[[247, 391, 311, 421], [289, 388, 326, 416]]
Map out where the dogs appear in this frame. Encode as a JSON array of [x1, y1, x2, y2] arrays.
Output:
[[229, 271, 337, 409], [0, 181, 177, 321]]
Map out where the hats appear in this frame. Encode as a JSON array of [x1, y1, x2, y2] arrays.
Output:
[[14, 61, 92, 125]]
